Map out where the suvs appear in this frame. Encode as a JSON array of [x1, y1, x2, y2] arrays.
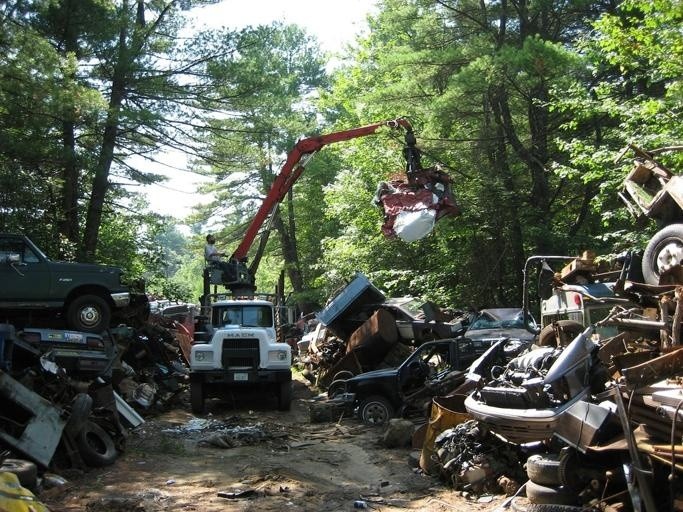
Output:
[[0, 232, 129, 333], [619, 144, 683, 288]]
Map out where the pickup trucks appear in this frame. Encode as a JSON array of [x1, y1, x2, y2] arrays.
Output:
[[342, 336, 484, 428]]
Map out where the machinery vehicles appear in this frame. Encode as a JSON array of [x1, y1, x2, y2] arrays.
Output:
[[185, 119, 460, 413]]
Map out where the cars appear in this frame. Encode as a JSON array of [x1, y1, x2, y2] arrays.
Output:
[[466, 306, 540, 364], [15, 318, 116, 385]]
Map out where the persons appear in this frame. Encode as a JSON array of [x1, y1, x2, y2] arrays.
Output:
[[203, 234, 237, 282]]
